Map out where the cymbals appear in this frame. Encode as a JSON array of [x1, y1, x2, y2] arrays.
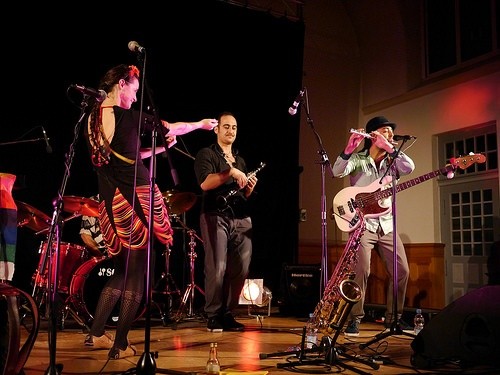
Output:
[[5, 199, 57, 237], [170, 226, 184, 230], [162, 188, 198, 216], [53, 196, 101, 218]]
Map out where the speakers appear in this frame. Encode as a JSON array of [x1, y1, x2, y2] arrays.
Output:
[[410, 284, 500, 369], [278, 264, 324, 317]]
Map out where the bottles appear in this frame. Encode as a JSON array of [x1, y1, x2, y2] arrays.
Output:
[[205, 343, 220, 375], [306, 313, 317, 349], [413, 309, 424, 335]]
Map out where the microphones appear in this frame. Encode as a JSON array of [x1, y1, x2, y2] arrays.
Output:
[[128, 41, 145, 53], [288, 88, 305, 115], [73, 84, 107, 102], [391, 134, 412, 142], [42, 126, 52, 153]]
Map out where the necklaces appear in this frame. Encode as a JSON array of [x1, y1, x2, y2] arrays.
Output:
[[226, 155, 233, 163]]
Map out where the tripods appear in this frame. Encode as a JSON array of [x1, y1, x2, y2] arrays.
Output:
[[255, 101, 418, 375], [170, 212, 207, 321]]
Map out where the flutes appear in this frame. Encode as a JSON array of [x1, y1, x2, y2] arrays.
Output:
[[348, 128, 399, 146], [213, 162, 265, 213]]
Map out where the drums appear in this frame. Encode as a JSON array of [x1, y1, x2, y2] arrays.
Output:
[[71, 254, 150, 328], [30, 239, 89, 296]]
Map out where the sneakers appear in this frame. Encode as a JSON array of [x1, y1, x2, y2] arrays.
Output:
[[83, 335, 115, 350], [385, 320, 412, 330], [109, 346, 138, 360], [344, 321, 361, 338], [207, 312, 245, 331]]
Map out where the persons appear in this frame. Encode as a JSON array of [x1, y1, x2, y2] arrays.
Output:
[[79, 194, 109, 257], [84, 66, 219, 359], [332, 116, 410, 338], [195, 112, 259, 333]]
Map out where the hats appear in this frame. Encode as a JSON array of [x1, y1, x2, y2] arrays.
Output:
[[364, 116, 395, 132]]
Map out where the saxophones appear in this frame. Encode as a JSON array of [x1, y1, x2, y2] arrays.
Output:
[[310, 197, 367, 336]]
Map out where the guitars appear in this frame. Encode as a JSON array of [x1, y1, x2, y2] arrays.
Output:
[[332, 151, 487, 232]]
